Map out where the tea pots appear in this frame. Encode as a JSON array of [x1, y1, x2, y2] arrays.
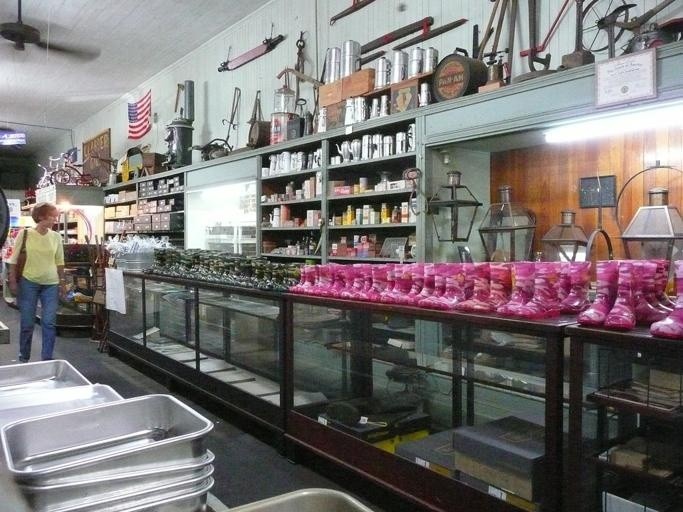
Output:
[[335, 137, 361, 163], [269, 207, 281, 228]]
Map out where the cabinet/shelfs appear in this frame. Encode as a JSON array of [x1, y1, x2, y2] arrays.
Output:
[[20, 202, 78, 244], [102, 180, 137, 243], [137, 168, 186, 248], [286, 293, 629, 510], [572, 316, 681, 508], [324, 109, 491, 276], [1, 255, 97, 339], [105, 268, 287, 438], [258, 134, 325, 267]]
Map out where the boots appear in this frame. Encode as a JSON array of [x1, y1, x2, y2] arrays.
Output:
[[288, 262, 590, 320], [576, 259, 683, 340], [141, 248, 301, 293]]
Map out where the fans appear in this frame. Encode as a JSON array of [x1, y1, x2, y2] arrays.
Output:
[[0, 0, 105, 64]]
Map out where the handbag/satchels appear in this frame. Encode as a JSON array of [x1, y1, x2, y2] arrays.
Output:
[[15, 230, 28, 283]]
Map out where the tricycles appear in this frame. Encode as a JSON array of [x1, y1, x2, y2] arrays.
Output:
[[34, 150, 101, 187]]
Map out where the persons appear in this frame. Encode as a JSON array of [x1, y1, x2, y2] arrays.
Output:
[[5, 201, 67, 363]]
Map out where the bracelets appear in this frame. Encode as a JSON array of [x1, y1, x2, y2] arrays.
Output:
[[60, 278, 66, 281], [7, 269, 16, 273]]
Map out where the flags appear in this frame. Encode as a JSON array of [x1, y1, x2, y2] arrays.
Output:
[[127, 89, 152, 139]]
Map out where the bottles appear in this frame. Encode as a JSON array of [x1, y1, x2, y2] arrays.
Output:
[[391, 205, 400, 222], [295, 235, 318, 255], [268, 147, 322, 175], [361, 123, 416, 162], [343, 80, 433, 129], [373, 46, 440, 91], [322, 40, 360, 86], [317, 105, 327, 133]]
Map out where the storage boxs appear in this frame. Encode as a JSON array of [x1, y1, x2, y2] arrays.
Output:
[[392, 425, 478, 481], [104, 189, 137, 235], [138, 212, 184, 231], [317, 78, 340, 111], [452, 413, 579, 499], [340, 68, 374, 102]]
